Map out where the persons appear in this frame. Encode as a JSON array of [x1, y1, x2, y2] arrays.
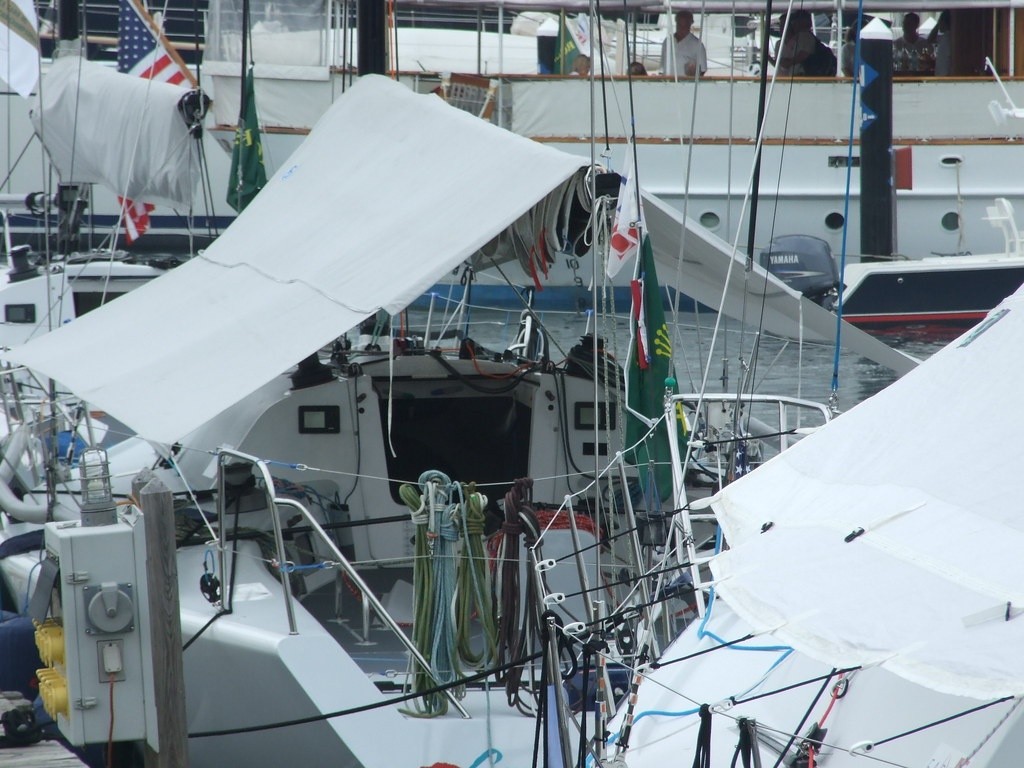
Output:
[[569, 54, 591, 76], [768, 12, 953, 77], [626, 62, 648, 78], [661, 11, 708, 80]]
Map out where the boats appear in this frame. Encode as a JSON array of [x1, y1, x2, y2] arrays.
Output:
[[0, 1, 1024, 306]]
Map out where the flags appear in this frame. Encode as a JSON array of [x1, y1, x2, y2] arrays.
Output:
[[116, 1, 192, 244], [226, 68, 268, 213], [607, 137, 650, 278], [554, 14, 583, 76], [625, 233, 691, 504]]
